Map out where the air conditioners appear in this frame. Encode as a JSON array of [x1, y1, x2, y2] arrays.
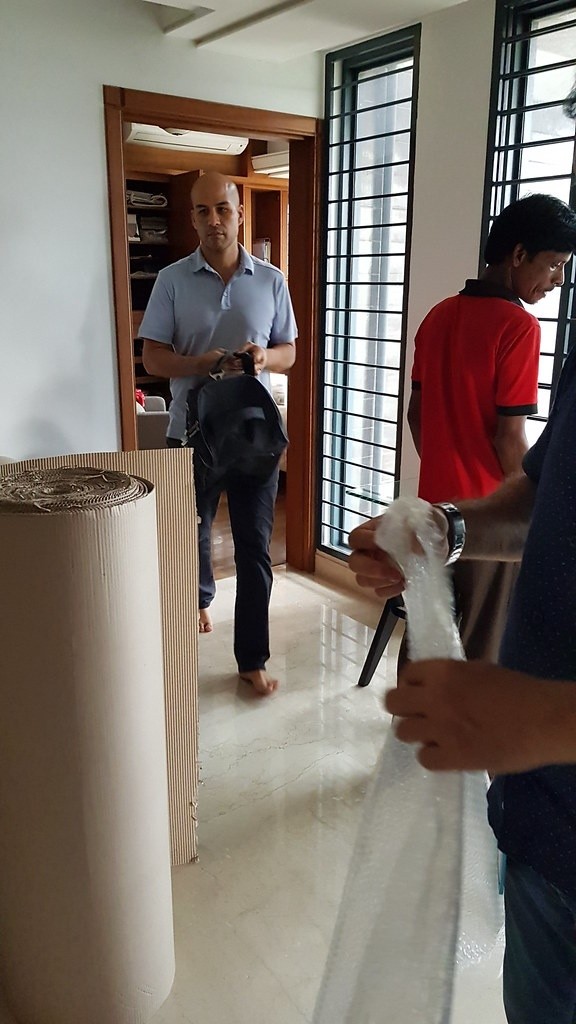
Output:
[[123, 121, 249, 156]]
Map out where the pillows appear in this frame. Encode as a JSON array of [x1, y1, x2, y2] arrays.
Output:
[[135, 388, 146, 415]]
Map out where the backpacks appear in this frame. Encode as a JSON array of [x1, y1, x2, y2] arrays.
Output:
[[185, 351, 289, 496]]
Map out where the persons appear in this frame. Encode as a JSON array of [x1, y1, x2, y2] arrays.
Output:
[[138, 171, 298, 695], [346, 81, 576, 1024]]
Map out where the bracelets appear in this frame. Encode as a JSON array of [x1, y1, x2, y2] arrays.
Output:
[[431, 502, 466, 566]]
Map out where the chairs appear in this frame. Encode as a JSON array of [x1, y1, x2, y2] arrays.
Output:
[[133, 387, 174, 451]]
